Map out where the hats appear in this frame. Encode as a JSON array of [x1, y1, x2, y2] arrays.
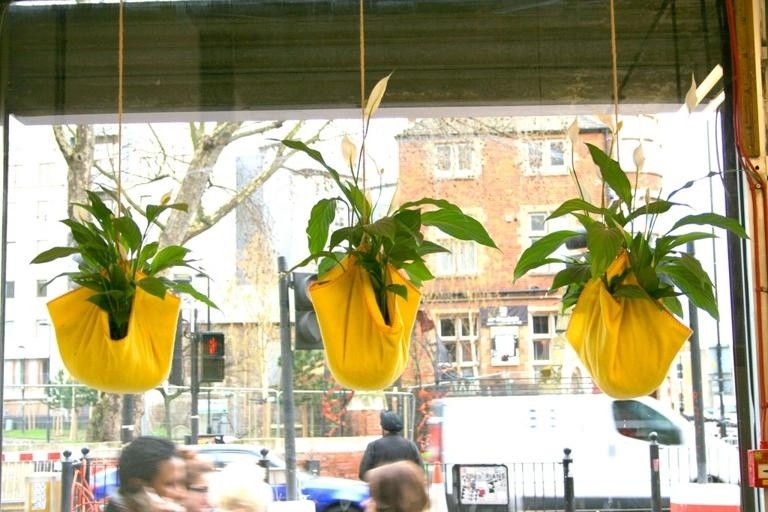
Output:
[[379, 411, 404, 432]]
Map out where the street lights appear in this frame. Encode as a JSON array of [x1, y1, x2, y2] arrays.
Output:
[[196, 274, 212, 331]]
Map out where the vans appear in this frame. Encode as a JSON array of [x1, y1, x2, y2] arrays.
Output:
[[427, 390, 740, 490]]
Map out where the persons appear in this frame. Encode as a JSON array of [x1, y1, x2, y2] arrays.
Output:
[[171, 447, 217, 512], [101, 436, 187, 512], [358, 409, 426, 482], [359, 459, 429, 512]]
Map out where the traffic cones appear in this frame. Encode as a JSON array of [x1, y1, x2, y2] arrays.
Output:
[[430, 461, 443, 484]]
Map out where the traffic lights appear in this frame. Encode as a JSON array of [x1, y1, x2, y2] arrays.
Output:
[[287, 266, 333, 349], [199, 332, 224, 383]]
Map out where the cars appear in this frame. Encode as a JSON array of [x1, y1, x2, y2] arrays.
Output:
[[678, 395, 737, 450], [77, 438, 377, 511]]
